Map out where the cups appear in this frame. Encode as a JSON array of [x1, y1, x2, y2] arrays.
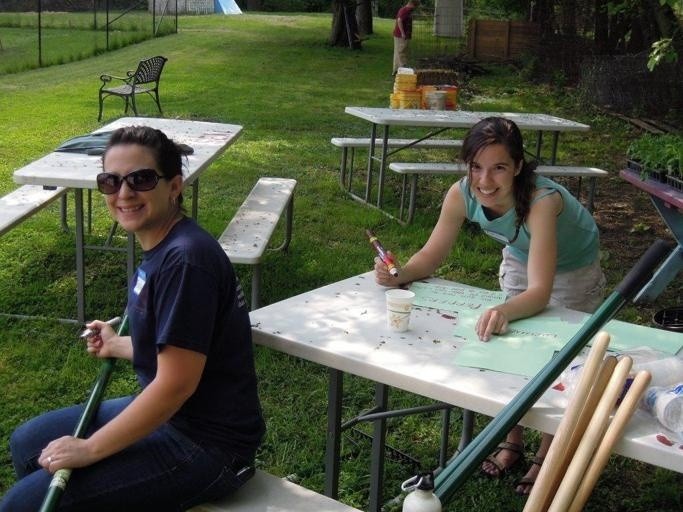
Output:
[[643, 385, 683, 434], [384, 289, 415, 333]]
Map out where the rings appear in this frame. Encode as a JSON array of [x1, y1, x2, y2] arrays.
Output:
[[46, 455, 58, 466]]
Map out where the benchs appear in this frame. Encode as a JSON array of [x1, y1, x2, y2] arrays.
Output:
[[219, 176, 297, 310], [0, 182, 69, 319], [384, 161, 608, 230], [198, 471, 360, 512], [331, 137, 528, 192]]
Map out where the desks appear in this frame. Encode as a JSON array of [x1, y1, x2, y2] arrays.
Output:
[[12, 115, 244, 325], [345, 106, 591, 211], [249, 269, 682, 512]]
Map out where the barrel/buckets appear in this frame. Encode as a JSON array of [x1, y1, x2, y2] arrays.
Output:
[[390, 74, 457, 110]]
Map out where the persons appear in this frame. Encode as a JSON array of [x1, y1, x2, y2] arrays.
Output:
[[371, 115, 608, 496], [0, 125, 266, 512], [390, 1, 418, 78]]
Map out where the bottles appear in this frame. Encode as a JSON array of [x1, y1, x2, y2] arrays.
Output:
[[401, 471, 443, 512]]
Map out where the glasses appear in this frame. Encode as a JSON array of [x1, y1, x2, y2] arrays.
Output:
[[95, 168, 167, 195]]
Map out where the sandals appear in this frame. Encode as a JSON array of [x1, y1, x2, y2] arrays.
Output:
[[512, 454, 544, 495], [479, 438, 526, 478]]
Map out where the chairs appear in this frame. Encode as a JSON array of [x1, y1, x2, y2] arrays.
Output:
[[96, 55, 167, 122]]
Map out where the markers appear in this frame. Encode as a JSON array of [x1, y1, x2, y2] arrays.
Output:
[[366, 228, 398, 278], [80, 316, 121, 338]]
